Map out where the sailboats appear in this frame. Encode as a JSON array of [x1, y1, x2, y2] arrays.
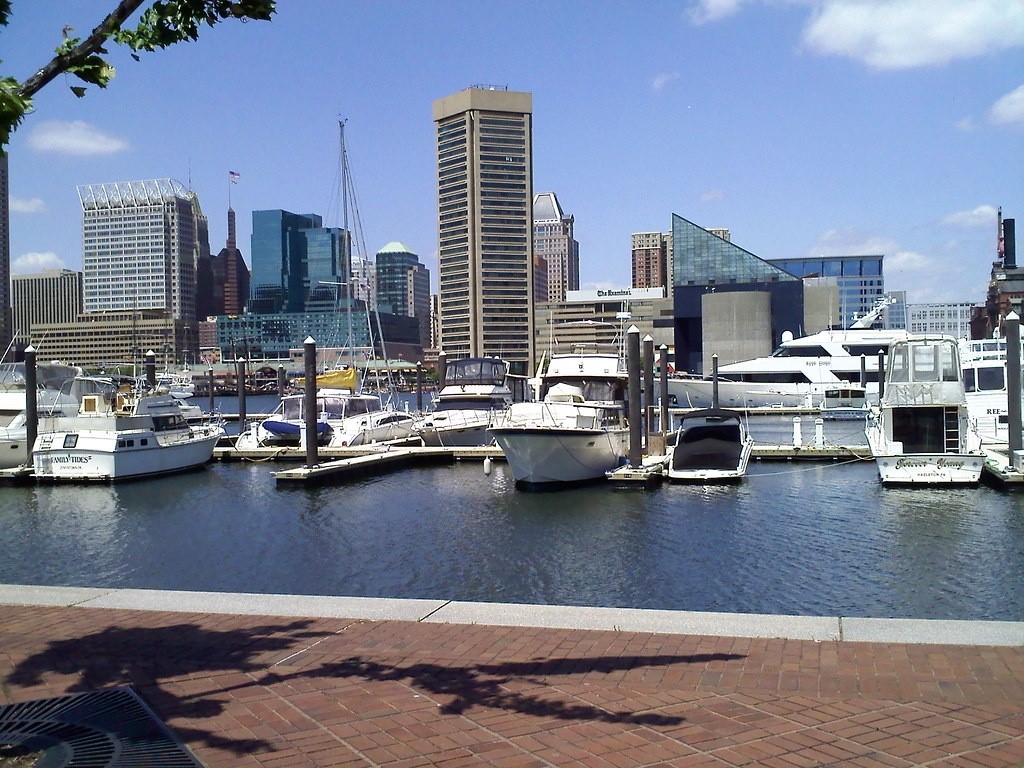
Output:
[[119, 295, 194, 401], [258, 111, 412, 445]]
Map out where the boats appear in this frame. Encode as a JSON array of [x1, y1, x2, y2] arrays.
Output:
[[642, 269, 1024, 421], [484, 306, 662, 491], [0, 328, 223, 482], [865, 337, 988, 490], [416, 356, 534, 451], [667, 407, 755, 485]]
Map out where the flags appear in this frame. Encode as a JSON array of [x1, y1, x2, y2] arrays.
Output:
[[669, 364, 677, 373], [230, 170, 240, 184]]
